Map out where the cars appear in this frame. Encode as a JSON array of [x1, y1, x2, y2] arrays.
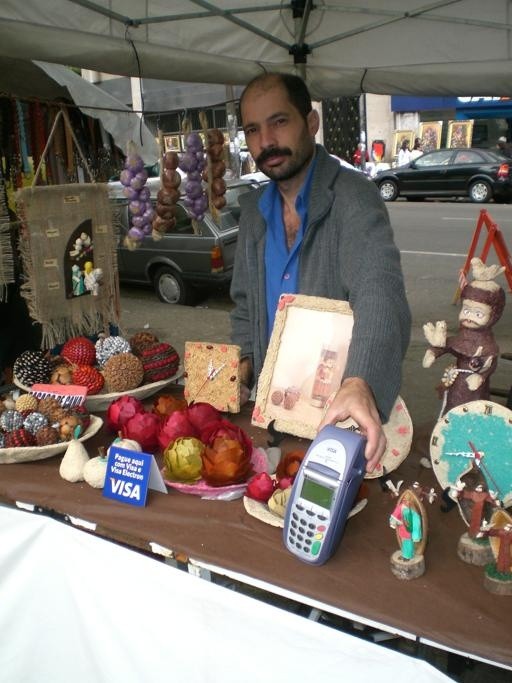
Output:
[[106, 170, 271, 308], [240, 155, 369, 191], [370, 147, 512, 203]]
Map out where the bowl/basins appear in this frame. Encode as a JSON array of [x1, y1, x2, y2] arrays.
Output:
[[13, 363, 186, 412]]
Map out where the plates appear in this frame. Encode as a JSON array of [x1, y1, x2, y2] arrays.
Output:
[[1, 412, 105, 464]]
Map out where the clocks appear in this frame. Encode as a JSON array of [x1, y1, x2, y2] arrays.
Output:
[[428, 397, 512, 509], [183, 340, 243, 415], [321, 386, 414, 478]]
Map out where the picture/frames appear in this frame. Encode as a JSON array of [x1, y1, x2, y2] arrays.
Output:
[[417, 120, 444, 153], [162, 127, 249, 153], [249, 292, 357, 439], [392, 129, 416, 159], [446, 119, 475, 148]]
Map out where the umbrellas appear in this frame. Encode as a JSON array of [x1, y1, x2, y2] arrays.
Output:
[[0, 0, 512, 105]]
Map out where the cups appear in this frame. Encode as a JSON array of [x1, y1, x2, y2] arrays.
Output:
[[309, 343, 336, 407]]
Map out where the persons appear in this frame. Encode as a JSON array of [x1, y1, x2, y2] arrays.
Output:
[[413, 136, 423, 151], [397, 138, 412, 166], [421, 261, 506, 469], [409, 143, 424, 162], [352, 141, 369, 168], [495, 139, 509, 155], [221, 70, 413, 476]]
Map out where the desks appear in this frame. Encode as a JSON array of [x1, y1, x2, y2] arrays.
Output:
[[0, 381, 512, 683]]
